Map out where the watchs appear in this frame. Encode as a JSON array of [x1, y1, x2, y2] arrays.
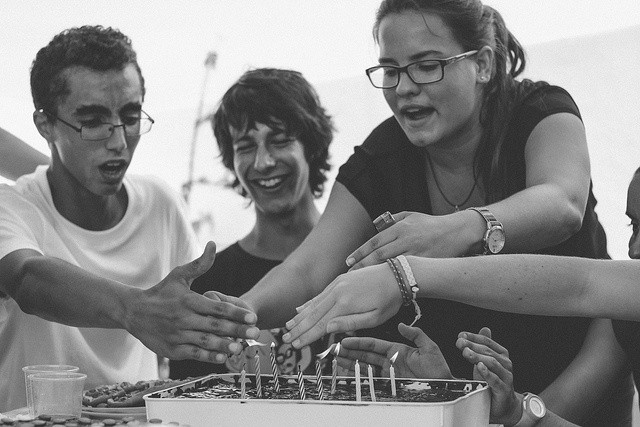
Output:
[[513, 391, 547, 426], [465, 205, 506, 256]]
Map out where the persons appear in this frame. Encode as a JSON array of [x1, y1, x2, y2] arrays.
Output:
[[0, 24, 260, 413], [168, 65, 334, 384], [203, 2, 633, 427], [282, 164, 639, 427]]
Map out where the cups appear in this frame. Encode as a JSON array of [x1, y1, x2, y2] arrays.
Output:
[[29, 374, 82, 426], [21, 363, 81, 416]]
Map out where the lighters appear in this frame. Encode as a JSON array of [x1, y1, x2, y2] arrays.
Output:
[[372, 210, 397, 232]]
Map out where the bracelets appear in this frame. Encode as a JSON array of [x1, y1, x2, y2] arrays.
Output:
[[386, 257, 411, 307], [396, 253, 419, 301]]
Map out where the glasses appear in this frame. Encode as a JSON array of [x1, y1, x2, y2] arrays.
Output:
[[39, 109, 154, 141], [365, 49, 478, 89]]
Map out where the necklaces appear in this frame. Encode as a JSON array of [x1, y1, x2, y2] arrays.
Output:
[[419, 144, 483, 214]]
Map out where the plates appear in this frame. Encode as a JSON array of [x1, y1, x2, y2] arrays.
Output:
[[143, 374, 491, 425], [73, 378, 200, 422]]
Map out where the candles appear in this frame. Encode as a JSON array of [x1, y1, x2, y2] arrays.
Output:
[[367, 363, 377, 401], [297, 364, 306, 399], [315, 359, 324, 399], [354, 358, 362, 401], [240, 363, 247, 398], [269, 340, 280, 392], [389, 349, 399, 395], [330, 357, 337, 394], [253, 350, 264, 397]]
